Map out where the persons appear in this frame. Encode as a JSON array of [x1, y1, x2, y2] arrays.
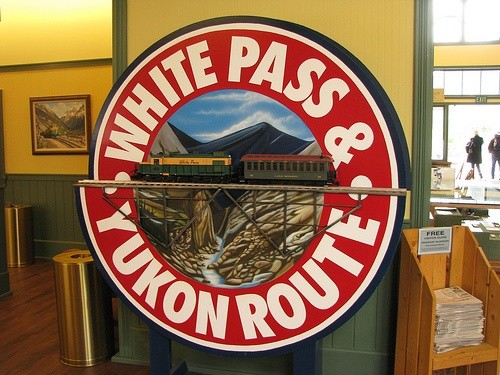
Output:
[[465, 130, 484, 179], [488, 132, 500, 179]]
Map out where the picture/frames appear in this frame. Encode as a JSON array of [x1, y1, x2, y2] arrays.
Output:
[[29, 94, 92, 156]]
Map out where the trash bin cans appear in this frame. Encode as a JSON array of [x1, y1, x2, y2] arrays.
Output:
[[52, 250, 112, 368], [3, 205, 34, 268]]
